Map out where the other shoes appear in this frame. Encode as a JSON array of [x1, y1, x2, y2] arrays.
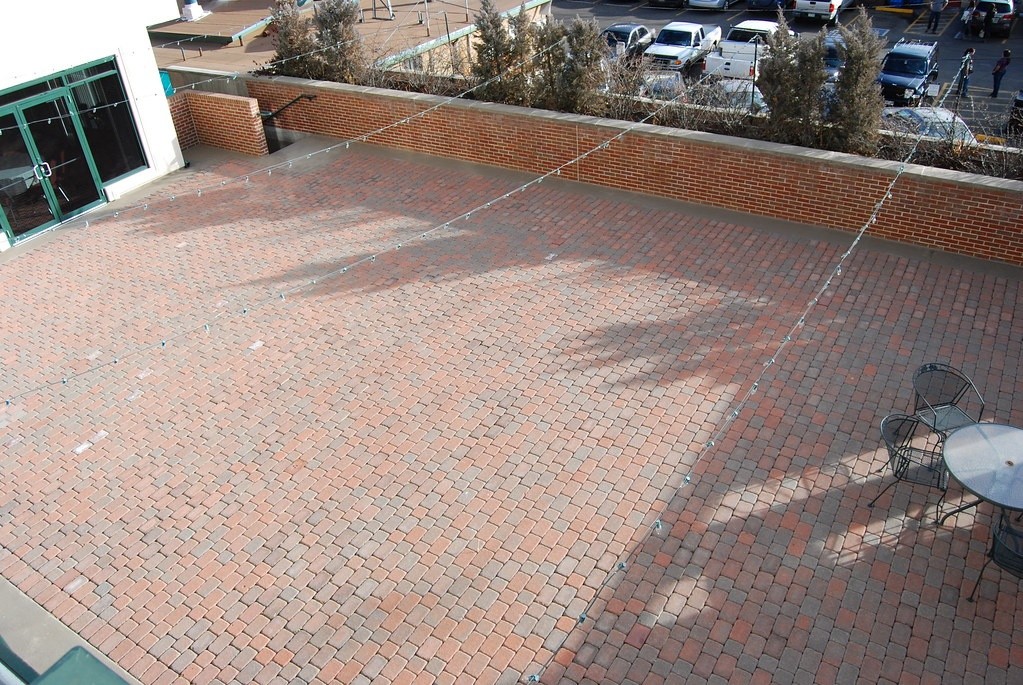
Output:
[[962, 95, 969, 98]]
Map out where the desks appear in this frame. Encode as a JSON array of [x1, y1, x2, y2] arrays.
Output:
[[940, 423, 1023, 557], [0, 151, 70, 225]]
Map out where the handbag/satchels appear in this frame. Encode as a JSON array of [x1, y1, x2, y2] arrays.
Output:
[[969, 63, 973, 73], [979, 29, 984, 37]]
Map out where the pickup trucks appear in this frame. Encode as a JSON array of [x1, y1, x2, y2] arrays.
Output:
[[642, 21, 722, 76], [702, 20, 798, 80]]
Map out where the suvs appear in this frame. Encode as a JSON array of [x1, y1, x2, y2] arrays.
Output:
[[969, 0, 1019, 38], [875, 37, 939, 106]]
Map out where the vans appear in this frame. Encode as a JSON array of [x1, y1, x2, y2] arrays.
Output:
[[791, 0, 853, 27]]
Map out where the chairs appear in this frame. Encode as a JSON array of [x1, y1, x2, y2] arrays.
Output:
[[890, 363, 985, 472], [966, 514, 1023, 603], [867, 414, 949, 525], [50, 150, 73, 202]]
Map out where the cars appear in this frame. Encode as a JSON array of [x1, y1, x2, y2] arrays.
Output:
[[747, 0, 794, 15], [595, 22, 655, 64], [882, 106, 978, 145], [689, 0, 740, 10], [633, 71, 688, 102], [813, 28, 850, 120], [649, 0, 689, 8], [694, 80, 771, 113]]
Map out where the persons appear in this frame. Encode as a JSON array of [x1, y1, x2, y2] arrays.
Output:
[[983, 3, 997, 40], [955, 48, 976, 99], [925, 0, 949, 34], [961, 0, 975, 41], [991, 49, 1011, 98]]
